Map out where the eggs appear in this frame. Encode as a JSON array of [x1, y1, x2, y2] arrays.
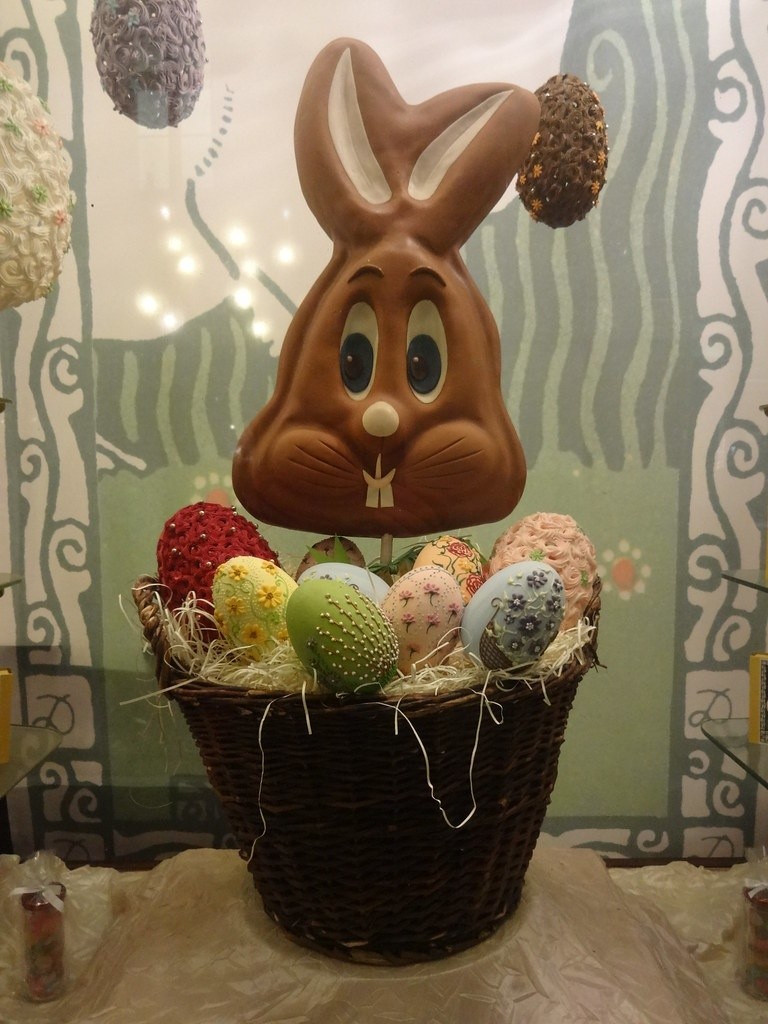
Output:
[[211, 533, 564, 695]]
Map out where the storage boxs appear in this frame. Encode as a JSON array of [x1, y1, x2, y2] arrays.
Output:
[[131, 575, 602, 968]]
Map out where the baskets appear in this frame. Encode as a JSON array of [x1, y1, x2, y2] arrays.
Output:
[[129, 572, 603, 967]]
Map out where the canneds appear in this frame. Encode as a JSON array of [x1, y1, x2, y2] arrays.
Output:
[[21, 882, 66, 1003]]
